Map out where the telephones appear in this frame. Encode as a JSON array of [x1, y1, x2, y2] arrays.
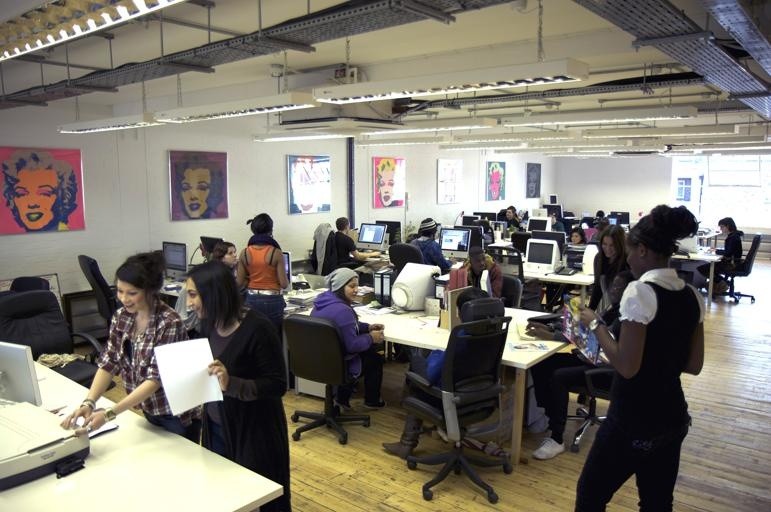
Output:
[[555, 265, 576, 275]]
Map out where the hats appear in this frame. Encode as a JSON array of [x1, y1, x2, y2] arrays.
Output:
[[420, 218, 437, 236], [325, 267, 360, 294]]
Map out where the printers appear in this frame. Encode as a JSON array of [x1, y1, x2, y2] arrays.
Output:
[[0, 402, 89, 493]]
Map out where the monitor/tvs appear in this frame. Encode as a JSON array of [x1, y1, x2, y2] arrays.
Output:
[[0, 342, 44, 406], [462, 215, 481, 226], [543, 204, 563, 219], [473, 212, 497, 221], [440, 228, 471, 258], [392, 262, 441, 310], [611, 211, 630, 225], [355, 222, 388, 253], [454, 226, 484, 251], [282, 251, 293, 294], [376, 221, 403, 245], [607, 217, 620, 227], [525, 238, 560, 274], [163, 240, 188, 280], [528, 218, 547, 230], [675, 234, 698, 253], [531, 208, 548, 217], [531, 229, 566, 260]]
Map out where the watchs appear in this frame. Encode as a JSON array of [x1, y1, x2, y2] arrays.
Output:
[[589, 315, 608, 332]]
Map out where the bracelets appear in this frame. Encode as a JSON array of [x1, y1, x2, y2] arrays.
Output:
[[79, 399, 101, 411], [101, 405, 120, 425]]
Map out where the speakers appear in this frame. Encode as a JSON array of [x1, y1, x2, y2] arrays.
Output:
[[383, 234, 390, 251]]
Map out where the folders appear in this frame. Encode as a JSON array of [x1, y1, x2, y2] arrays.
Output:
[[374, 270, 391, 307]]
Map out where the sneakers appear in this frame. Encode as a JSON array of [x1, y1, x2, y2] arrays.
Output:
[[334, 397, 352, 411], [528, 414, 550, 434], [383, 441, 413, 460], [532, 438, 567, 461], [362, 398, 388, 410]]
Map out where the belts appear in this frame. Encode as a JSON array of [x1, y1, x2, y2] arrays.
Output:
[[247, 288, 284, 296]]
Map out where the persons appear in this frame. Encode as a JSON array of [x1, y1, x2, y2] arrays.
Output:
[[532, 267, 631, 460], [3, 151, 78, 229], [589, 223, 629, 311], [174, 154, 226, 218], [382, 285, 490, 459], [477, 205, 605, 260], [240, 213, 291, 344], [490, 163, 509, 200], [697, 217, 743, 296], [210, 241, 237, 282], [529, 164, 542, 197], [291, 156, 332, 213], [377, 159, 403, 206], [178, 263, 296, 512], [320, 218, 381, 276], [310, 265, 387, 410], [408, 217, 457, 270], [456, 247, 503, 297], [574, 204, 709, 512], [60, 252, 200, 444]]
